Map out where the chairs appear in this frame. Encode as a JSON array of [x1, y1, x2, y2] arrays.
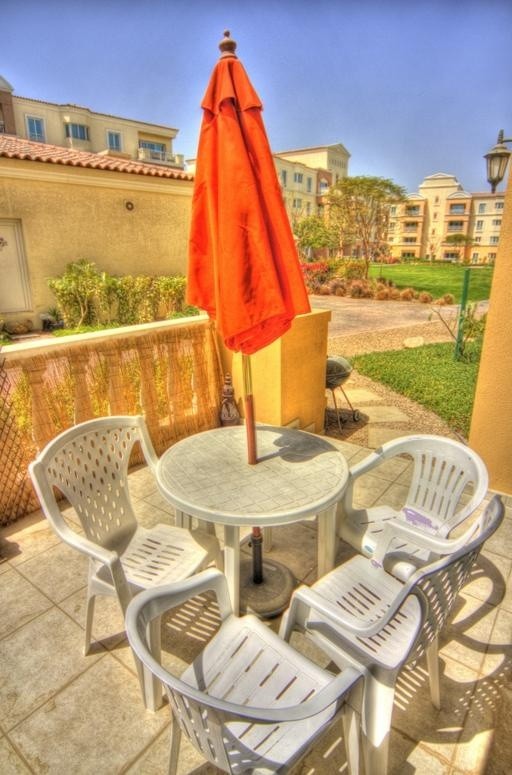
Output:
[[278, 494, 505, 775], [125, 569, 370, 775], [28, 415, 224, 711], [335, 434, 489, 583]]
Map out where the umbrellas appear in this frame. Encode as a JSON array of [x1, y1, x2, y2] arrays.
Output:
[[182, 26, 314, 581]]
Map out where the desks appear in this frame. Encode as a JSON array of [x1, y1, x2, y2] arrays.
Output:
[[155, 425, 349, 620]]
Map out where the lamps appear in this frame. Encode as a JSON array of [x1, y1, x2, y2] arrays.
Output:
[[482, 129, 512, 193]]
